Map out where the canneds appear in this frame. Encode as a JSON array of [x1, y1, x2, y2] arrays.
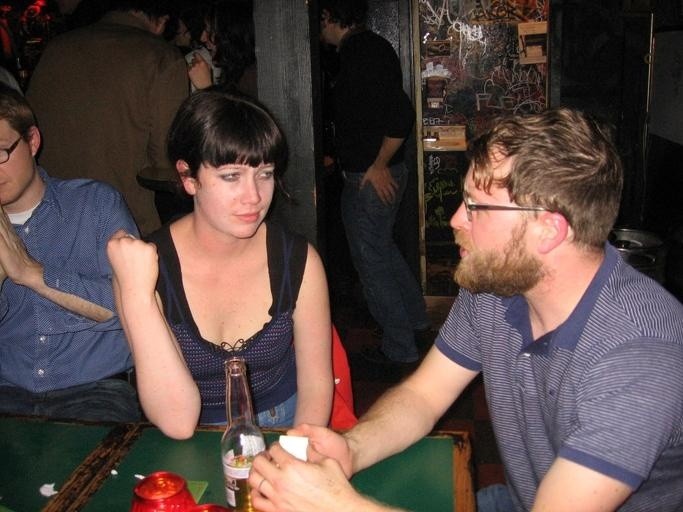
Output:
[[133, 472, 191, 512]]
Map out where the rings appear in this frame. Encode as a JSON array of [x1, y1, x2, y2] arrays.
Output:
[[256, 478, 268, 492]]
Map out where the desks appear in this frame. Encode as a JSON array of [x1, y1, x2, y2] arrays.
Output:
[[66, 422, 478, 512], [0, 412, 136, 512]]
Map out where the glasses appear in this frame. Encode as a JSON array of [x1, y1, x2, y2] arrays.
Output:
[[461, 192, 557, 220], [0, 136, 23, 164]]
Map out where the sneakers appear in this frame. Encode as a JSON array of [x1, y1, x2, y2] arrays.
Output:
[[357, 327, 430, 364]]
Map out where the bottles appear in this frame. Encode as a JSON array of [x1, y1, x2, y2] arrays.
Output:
[[131, 472, 197, 511], [220, 357, 268, 512]]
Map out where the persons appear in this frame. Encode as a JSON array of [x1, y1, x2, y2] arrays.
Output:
[[305, 3, 440, 377], [0, 77, 146, 431], [25, 1, 190, 240], [177, 10, 223, 93], [242, 102, 680, 511], [1, 67, 24, 96], [101, 81, 337, 443], [189, 1, 255, 97], [1, 1, 20, 80]]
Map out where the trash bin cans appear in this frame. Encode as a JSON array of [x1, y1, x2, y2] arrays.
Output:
[[609, 229, 668, 291]]
